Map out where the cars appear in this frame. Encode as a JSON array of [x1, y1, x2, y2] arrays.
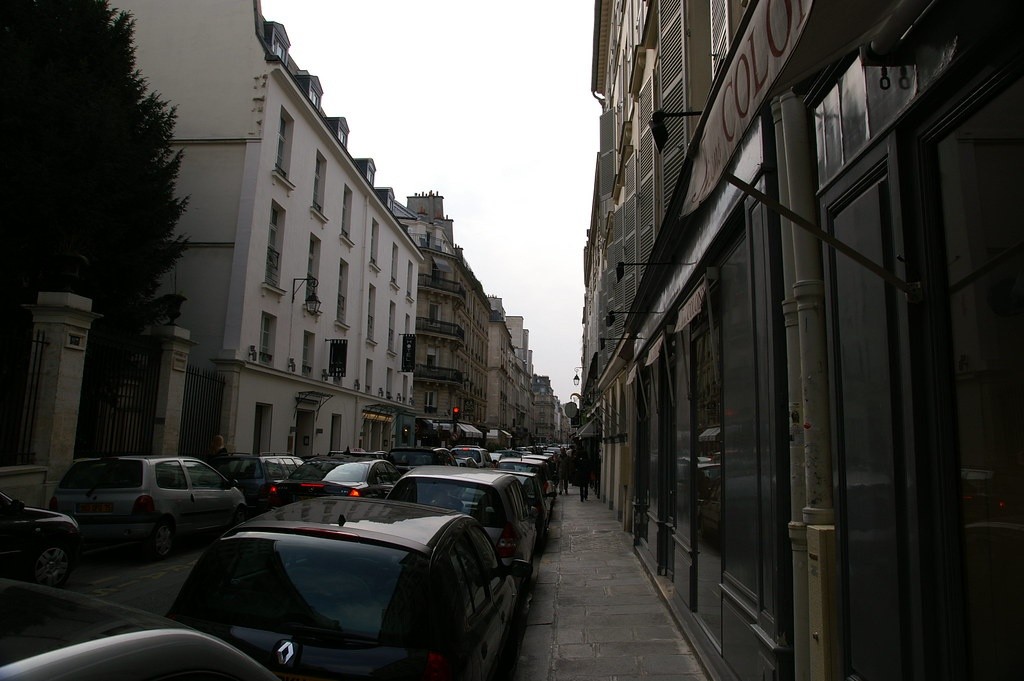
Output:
[[198, 451, 306, 504], [384, 464, 540, 589], [164, 496, 535, 681], [0, 576, 284, 681], [300, 443, 576, 539], [50, 452, 248, 559], [0, 491, 81, 586], [263, 453, 404, 507]]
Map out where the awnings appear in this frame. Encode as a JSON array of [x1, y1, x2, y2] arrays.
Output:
[[679, 0, 923, 304], [417, 419, 483, 439]]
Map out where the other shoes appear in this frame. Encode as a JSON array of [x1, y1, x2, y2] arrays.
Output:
[[565, 491, 568, 495], [559, 492, 562, 495]]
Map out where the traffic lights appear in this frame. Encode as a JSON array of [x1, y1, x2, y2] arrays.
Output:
[[453, 407, 459, 419]]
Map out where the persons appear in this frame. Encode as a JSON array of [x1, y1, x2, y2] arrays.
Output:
[[531, 444, 595, 502], [207, 435, 229, 468]]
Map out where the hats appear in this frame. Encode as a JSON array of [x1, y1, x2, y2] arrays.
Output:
[[560, 447, 566, 452]]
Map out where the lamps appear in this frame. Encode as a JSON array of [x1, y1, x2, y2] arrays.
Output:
[[322, 369, 328, 381], [249, 345, 256, 361], [606, 310, 657, 327], [409, 398, 413, 405], [397, 393, 401, 401], [305, 293, 321, 316], [616, 262, 693, 284], [379, 388, 383, 396], [52, 250, 89, 292], [648, 110, 702, 154], [288, 358, 295, 372], [600, 338, 642, 350], [163, 294, 187, 327], [354, 379, 360, 390]]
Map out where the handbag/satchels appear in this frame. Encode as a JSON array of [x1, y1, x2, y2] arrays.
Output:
[[572, 470, 582, 486]]
